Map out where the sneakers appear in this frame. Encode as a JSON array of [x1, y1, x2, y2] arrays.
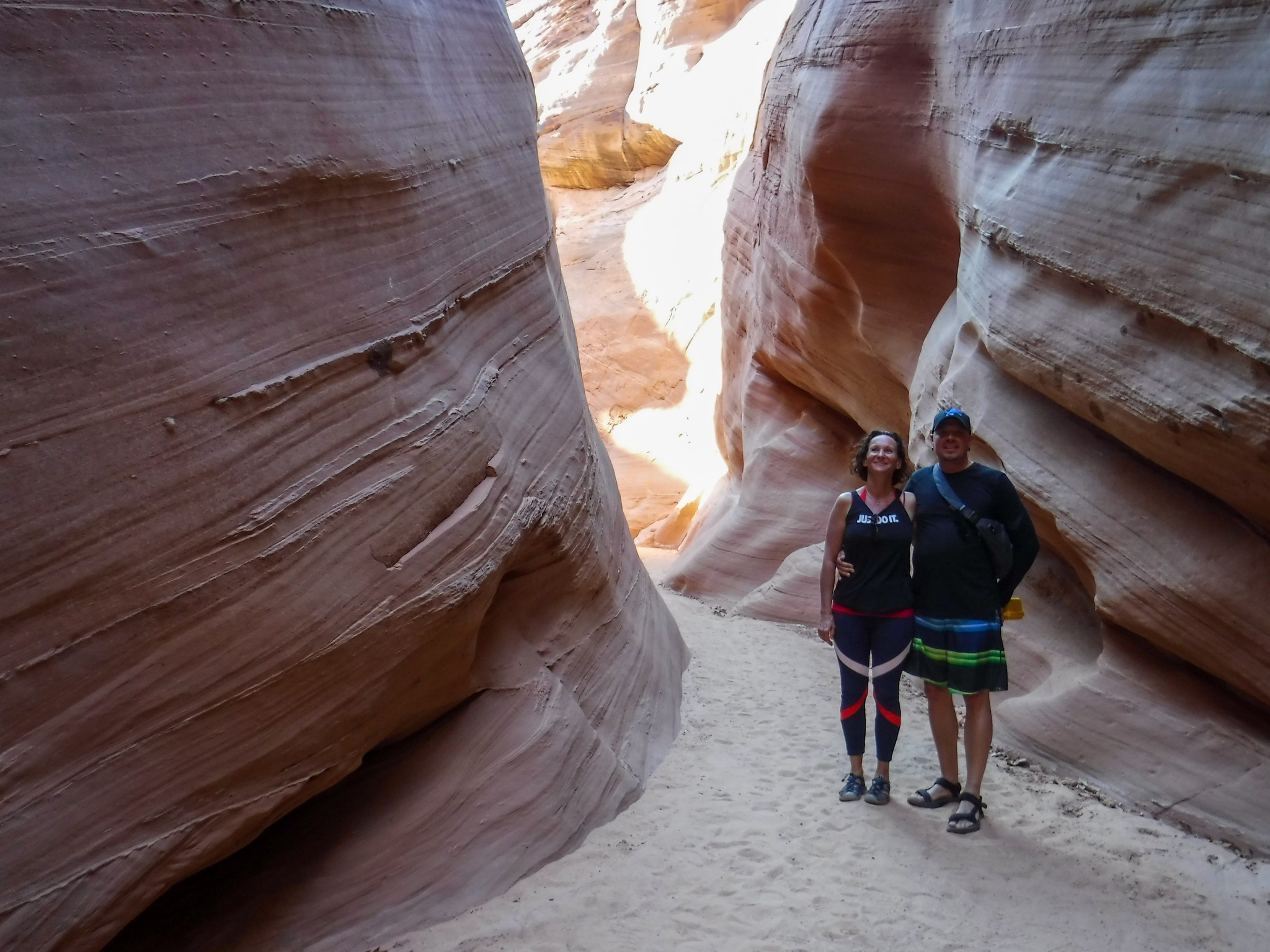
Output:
[[864, 776, 890, 804], [838, 772, 867, 801]]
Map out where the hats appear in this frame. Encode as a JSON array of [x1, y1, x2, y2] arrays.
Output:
[[932, 408, 971, 438]]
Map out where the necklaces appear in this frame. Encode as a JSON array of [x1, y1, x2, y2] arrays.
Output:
[[864, 486, 895, 506]]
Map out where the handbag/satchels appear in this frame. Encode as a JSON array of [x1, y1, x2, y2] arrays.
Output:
[[975, 517, 1014, 581]]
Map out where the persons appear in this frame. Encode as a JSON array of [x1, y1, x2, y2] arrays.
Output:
[[902, 407, 1040, 834], [818, 430, 915, 805]]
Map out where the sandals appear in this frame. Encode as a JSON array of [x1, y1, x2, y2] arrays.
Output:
[[907, 777, 961, 808], [946, 792, 987, 833]]
